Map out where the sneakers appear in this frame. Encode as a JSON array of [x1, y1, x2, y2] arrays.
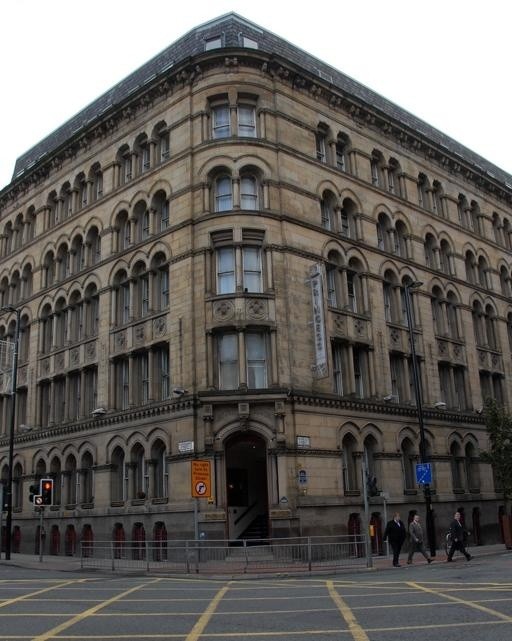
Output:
[[448, 556, 475, 562], [394, 564, 400, 566], [428, 559, 435, 563]]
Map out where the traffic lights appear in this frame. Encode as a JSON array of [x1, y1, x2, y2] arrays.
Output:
[[33, 478, 54, 507]]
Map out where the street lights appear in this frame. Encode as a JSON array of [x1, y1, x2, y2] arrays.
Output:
[[1, 303, 23, 563], [403, 280, 439, 558]]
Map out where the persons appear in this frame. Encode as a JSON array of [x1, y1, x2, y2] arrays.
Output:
[[445, 510, 476, 562], [382, 513, 406, 567], [407, 513, 435, 565]]
